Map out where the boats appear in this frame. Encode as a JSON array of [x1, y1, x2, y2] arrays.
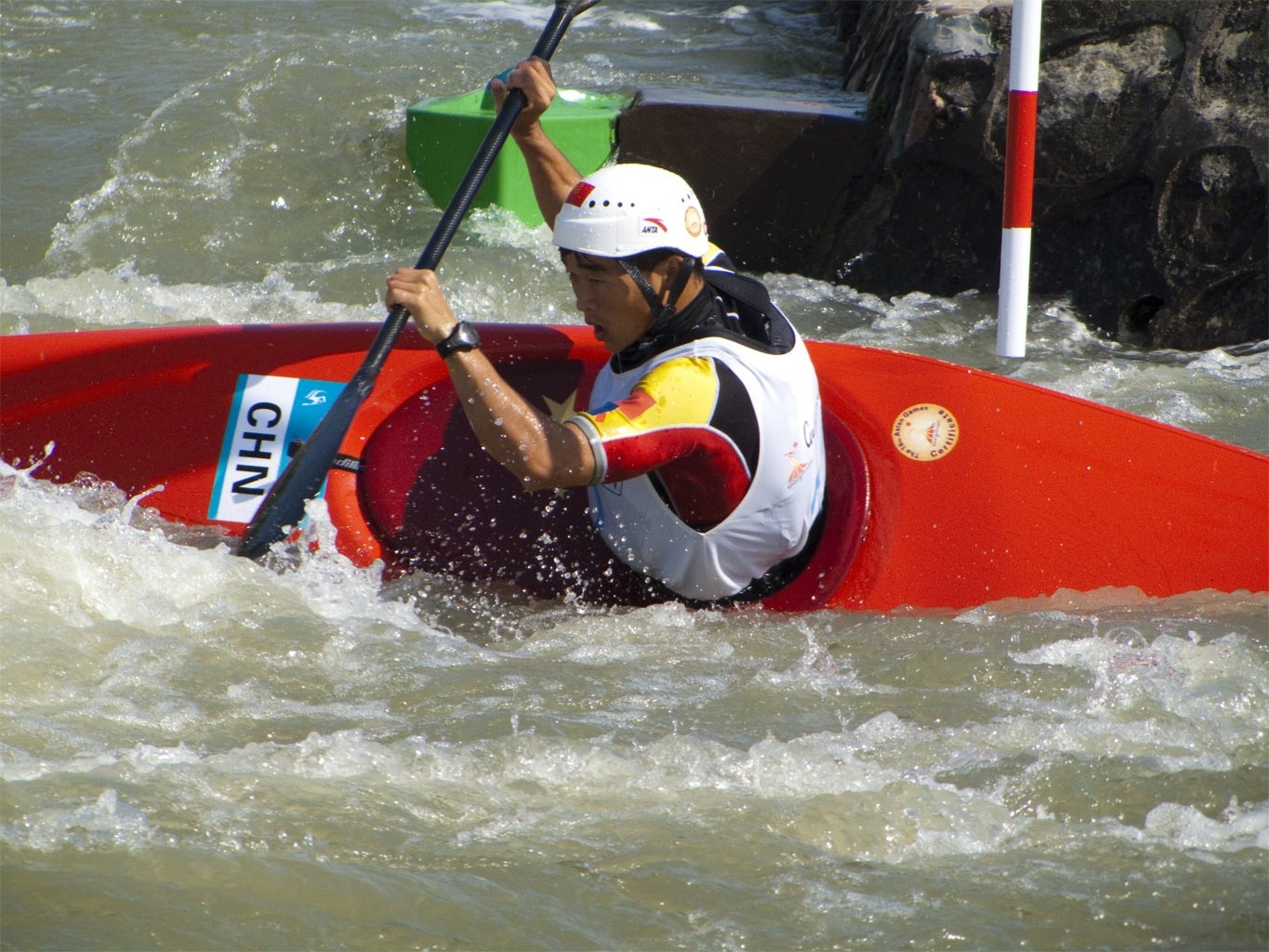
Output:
[[403, 80, 634, 229], [2, 315, 1268, 616]]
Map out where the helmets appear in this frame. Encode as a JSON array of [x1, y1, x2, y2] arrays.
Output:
[[552, 163, 708, 258]]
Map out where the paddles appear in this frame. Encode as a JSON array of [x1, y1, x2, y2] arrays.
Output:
[[236, 0, 602, 560]]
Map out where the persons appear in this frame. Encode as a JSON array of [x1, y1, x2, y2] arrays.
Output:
[[384, 54, 831, 614]]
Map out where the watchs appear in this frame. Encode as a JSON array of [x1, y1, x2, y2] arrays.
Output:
[[435, 320, 483, 360]]
